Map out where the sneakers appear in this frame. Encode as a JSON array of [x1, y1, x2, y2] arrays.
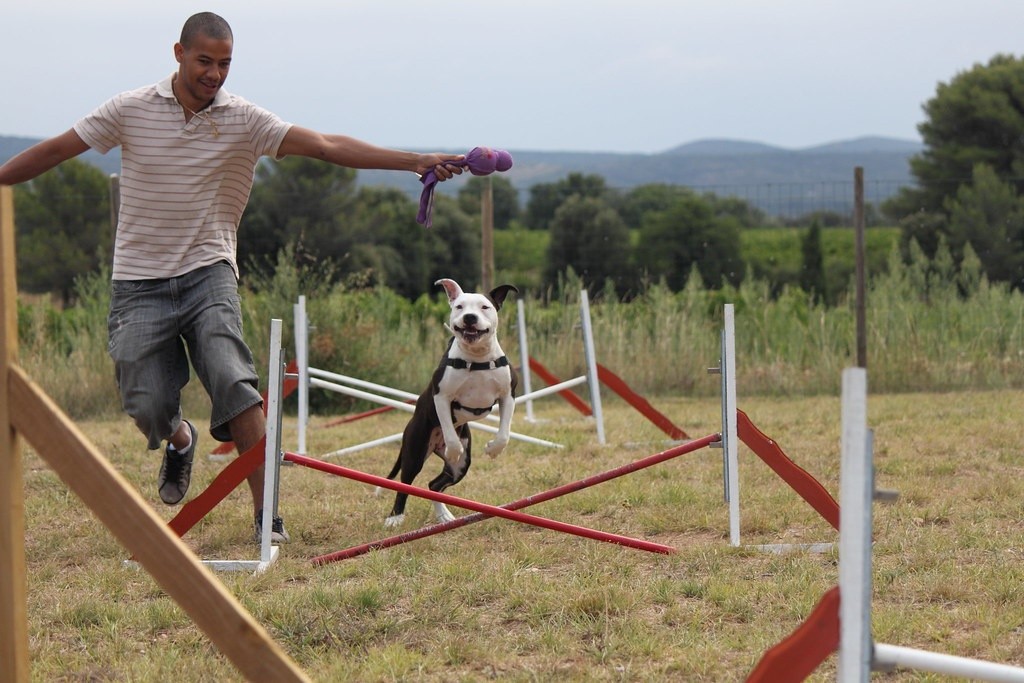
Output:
[[256, 509, 293, 546], [156, 419, 199, 505]]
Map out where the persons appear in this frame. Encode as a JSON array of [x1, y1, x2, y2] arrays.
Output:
[[1, 13, 469, 547]]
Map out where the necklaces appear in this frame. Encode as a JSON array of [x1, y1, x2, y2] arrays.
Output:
[[174, 79, 219, 139]]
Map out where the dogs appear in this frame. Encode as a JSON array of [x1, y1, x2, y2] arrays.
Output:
[[384, 278, 520, 529]]
